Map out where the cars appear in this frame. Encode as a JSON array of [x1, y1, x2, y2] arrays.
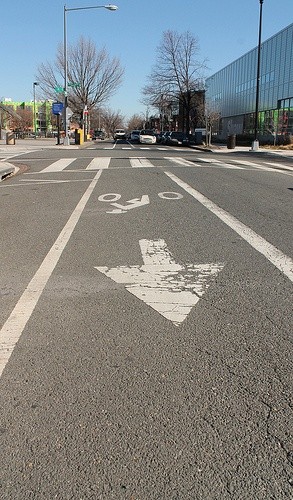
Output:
[[130, 130, 140, 142], [140, 129, 157, 145], [157, 131, 170, 144], [168, 131, 189, 146]]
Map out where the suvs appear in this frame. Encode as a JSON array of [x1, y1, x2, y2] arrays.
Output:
[[92, 131, 105, 141]]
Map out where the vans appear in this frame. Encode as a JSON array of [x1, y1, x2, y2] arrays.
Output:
[[113, 129, 127, 141]]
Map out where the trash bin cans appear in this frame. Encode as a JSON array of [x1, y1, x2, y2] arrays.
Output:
[[6, 132, 15, 145], [227, 135, 236, 149]]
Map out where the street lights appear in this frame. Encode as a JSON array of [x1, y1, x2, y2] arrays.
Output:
[[33, 81, 40, 139], [63, 5, 119, 146]]
